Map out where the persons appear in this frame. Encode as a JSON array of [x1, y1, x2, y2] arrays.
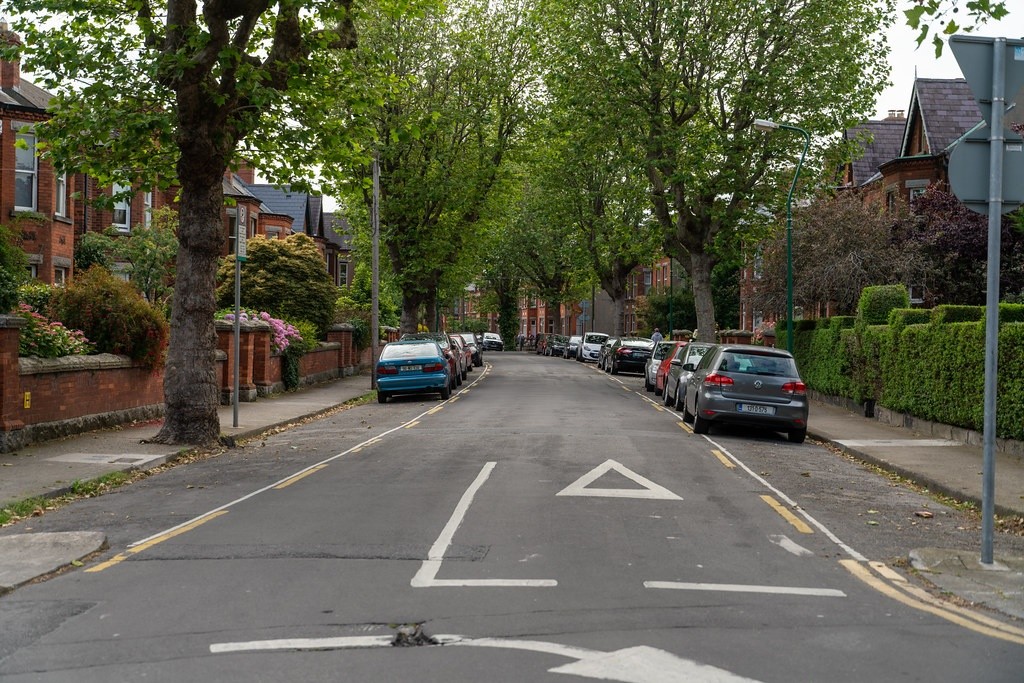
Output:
[[528, 331, 534, 350], [517, 331, 525, 351]]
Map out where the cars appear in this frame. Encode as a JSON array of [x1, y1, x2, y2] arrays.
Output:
[[679, 344, 810, 444], [660, 343, 713, 413], [398, 330, 505, 388], [653, 341, 692, 394], [563, 335, 582, 357], [542, 333, 567, 356], [642, 338, 676, 390], [374, 341, 453, 404], [601, 337, 655, 375], [576, 331, 609, 362], [597, 337, 619, 368], [536, 333, 560, 354]]
